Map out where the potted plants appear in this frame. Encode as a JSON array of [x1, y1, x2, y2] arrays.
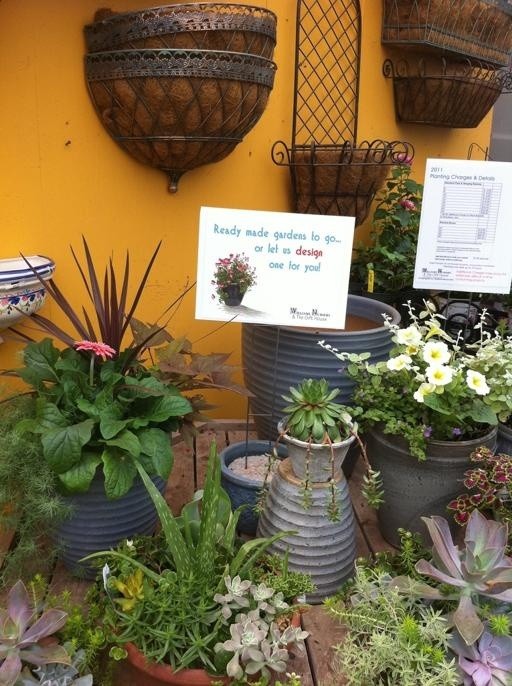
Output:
[[251, 377, 388, 522]]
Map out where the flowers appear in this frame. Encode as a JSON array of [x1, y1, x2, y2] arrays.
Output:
[[75, 433, 319, 686], [210, 252, 259, 304], [1, 233, 256, 505], [315, 294, 512, 461], [352, 150, 424, 292]]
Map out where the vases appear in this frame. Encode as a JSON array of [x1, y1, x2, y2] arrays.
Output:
[[222, 282, 247, 306], [90, 597, 301, 685], [43, 431, 172, 580], [222, 441, 286, 538], [363, 422, 500, 551]]
